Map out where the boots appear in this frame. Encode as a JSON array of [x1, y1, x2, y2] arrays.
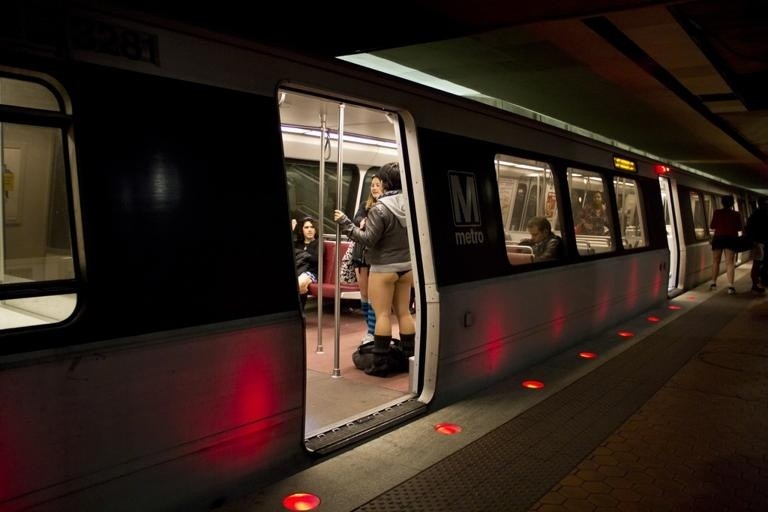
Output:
[[399, 333, 415, 372], [364, 335, 391, 377]]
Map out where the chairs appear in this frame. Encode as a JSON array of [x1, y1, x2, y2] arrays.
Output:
[[505, 226, 714, 266], [308, 240, 362, 299]]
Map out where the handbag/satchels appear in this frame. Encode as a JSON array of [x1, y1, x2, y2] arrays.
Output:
[[340, 240, 357, 286], [352, 338, 402, 372]]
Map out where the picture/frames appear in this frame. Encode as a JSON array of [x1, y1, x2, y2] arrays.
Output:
[[3, 142, 27, 224]]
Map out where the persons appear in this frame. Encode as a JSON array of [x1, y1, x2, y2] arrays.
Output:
[[349, 169, 384, 347], [578, 191, 608, 236], [517, 239, 534, 254], [711, 194, 743, 294], [527, 215, 563, 263], [335, 161, 416, 376], [294, 216, 328, 310], [601, 200, 607, 211], [750, 196, 768, 295]]
